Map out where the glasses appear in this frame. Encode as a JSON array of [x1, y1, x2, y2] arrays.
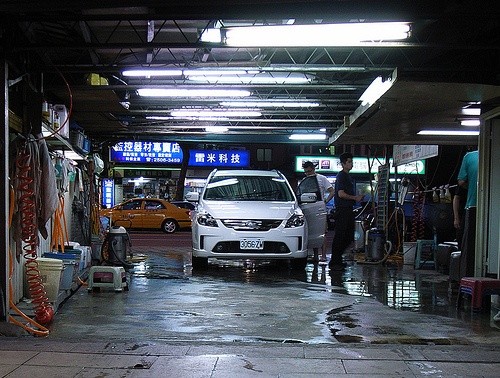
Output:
[[304, 169, 313, 174]]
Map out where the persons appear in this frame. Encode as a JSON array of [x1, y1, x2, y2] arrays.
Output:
[[453, 187, 467, 249], [328, 153, 365, 270], [301, 161, 335, 262], [458, 147, 478, 300]]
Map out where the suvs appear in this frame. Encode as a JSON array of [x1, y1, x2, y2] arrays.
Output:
[[184, 169, 327, 271]]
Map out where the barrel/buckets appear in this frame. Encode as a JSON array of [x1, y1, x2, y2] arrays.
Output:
[[437, 242, 459, 273], [403, 242, 417, 265], [26, 257, 63, 303], [450, 251, 462, 280], [42, 253, 76, 290], [57, 248, 81, 281]]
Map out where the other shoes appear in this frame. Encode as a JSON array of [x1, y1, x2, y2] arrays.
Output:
[[329, 262, 347, 268]]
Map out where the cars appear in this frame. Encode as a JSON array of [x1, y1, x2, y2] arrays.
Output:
[[98, 197, 192, 233]]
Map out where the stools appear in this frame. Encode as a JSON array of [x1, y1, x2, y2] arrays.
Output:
[[413, 240, 438, 271], [88, 266, 130, 293], [457, 277, 500, 313]]
